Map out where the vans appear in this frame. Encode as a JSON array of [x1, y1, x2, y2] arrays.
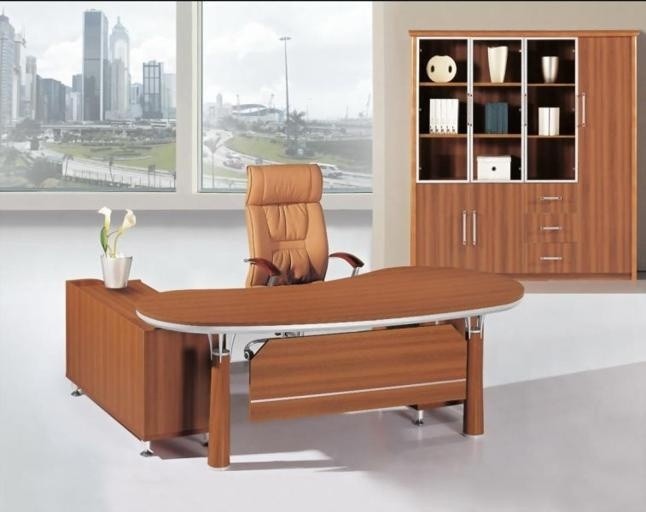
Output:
[[320, 163, 342, 177]]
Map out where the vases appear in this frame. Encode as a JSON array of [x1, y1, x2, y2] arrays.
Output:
[[99, 254, 133, 288]]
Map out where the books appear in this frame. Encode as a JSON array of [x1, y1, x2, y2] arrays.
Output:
[[427, 98, 460, 135], [538, 107, 549, 136], [484, 101, 510, 135], [549, 107, 560, 136]]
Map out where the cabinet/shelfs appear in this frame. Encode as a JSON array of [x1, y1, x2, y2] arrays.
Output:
[[406, 27, 639, 283], [62, 278, 220, 457]]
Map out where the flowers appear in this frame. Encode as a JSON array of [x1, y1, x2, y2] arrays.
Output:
[[97, 205, 136, 256]]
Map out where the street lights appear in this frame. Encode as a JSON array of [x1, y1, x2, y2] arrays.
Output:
[[280, 34, 291, 140]]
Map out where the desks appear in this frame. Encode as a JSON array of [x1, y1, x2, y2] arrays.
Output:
[[134, 265, 523, 473]]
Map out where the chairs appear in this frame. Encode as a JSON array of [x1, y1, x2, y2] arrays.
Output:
[[241, 161, 364, 290]]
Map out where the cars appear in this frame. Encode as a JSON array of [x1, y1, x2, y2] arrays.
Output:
[[225, 151, 239, 157], [223, 159, 242, 168]]
